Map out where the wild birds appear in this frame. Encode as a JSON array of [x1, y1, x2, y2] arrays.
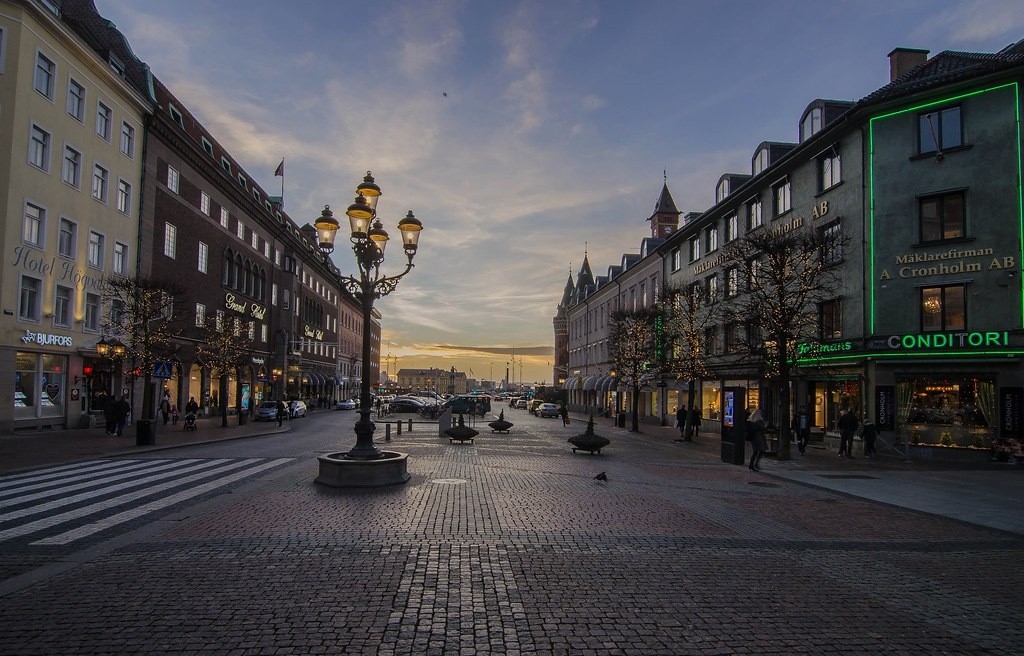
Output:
[[593, 472, 608, 483]]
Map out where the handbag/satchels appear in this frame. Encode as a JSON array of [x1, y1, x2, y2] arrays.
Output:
[[673, 420, 679, 429], [566, 416, 570, 424]]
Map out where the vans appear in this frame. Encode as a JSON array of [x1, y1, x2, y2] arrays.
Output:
[[443, 395, 492, 413], [420, 391, 446, 401]]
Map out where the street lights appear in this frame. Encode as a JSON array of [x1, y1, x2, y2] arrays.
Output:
[[313, 170, 423, 487], [96, 331, 126, 397]]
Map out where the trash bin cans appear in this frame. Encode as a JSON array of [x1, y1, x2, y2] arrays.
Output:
[[136, 419, 156, 446], [764, 425, 779, 457], [617, 413, 625, 427]]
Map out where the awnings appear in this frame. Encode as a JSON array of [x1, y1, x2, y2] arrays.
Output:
[[302, 373, 328, 386], [562, 375, 617, 391]]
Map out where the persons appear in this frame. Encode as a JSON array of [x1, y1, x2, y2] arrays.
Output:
[[158, 395, 179, 425], [289, 400, 295, 416], [310, 395, 331, 411], [792, 405, 811, 456], [837, 408, 858, 457], [103, 394, 130, 437], [184, 397, 198, 426], [247, 397, 254, 418], [275, 400, 285, 427], [859, 420, 880, 458], [676, 404, 688, 437], [691, 406, 701, 437], [559, 406, 567, 427], [745, 407, 769, 472], [511, 399, 514, 407]]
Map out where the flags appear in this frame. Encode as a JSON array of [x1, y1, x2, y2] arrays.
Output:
[[275, 157, 284, 177]]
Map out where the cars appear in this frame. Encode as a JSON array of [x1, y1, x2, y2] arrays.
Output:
[[255, 401, 307, 421], [336, 393, 397, 410], [388, 396, 448, 414], [510, 396, 560, 418]]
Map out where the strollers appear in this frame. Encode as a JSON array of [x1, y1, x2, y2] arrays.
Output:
[[183, 413, 197, 432]]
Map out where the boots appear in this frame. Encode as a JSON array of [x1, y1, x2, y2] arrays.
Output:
[[749, 455, 762, 471]]
